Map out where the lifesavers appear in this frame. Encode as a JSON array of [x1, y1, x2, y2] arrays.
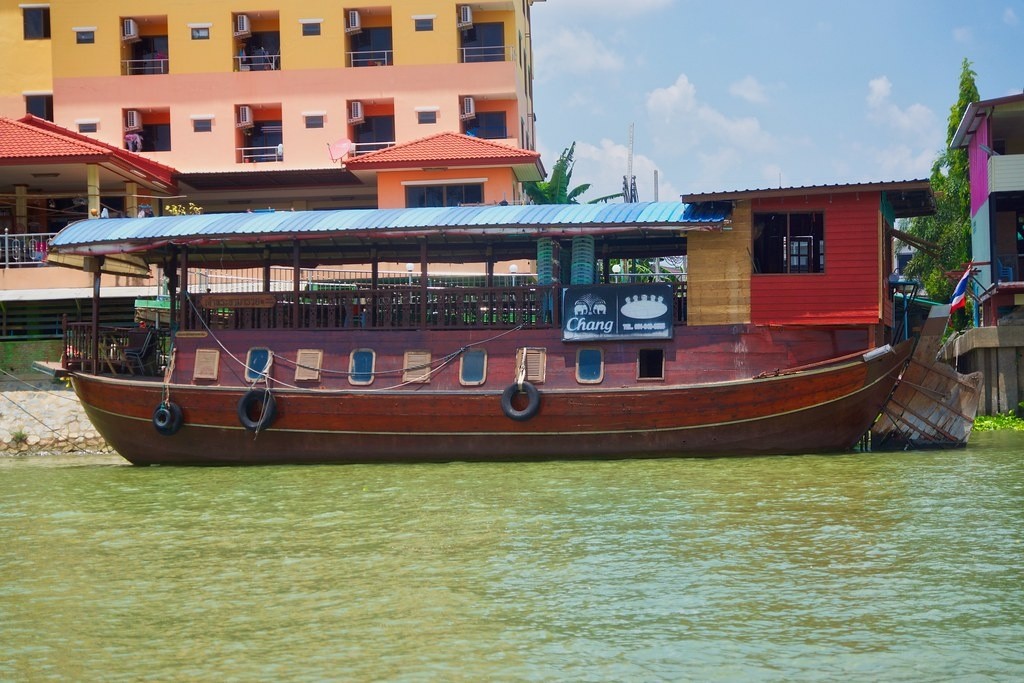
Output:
[[152, 402, 184, 436], [153, 408, 171, 429], [501, 382, 541, 421], [236, 387, 278, 430]]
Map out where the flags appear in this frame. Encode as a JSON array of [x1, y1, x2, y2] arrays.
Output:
[[949, 261, 974, 328]]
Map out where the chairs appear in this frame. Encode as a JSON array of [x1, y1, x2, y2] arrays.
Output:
[[996, 256, 1015, 283], [343, 301, 366, 328], [121, 331, 155, 373]]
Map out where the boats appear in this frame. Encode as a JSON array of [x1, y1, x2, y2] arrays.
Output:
[[47, 201, 921, 467]]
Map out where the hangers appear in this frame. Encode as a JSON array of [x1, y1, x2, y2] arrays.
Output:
[[72, 194, 88, 204]]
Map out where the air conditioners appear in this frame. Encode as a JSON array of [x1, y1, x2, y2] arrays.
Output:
[[352, 102, 363, 119], [461, 6, 473, 23], [128, 110, 144, 129], [464, 97, 475, 116], [239, 106, 254, 122], [238, 15, 251, 32], [124, 19, 139, 37], [349, 11, 362, 29]]
